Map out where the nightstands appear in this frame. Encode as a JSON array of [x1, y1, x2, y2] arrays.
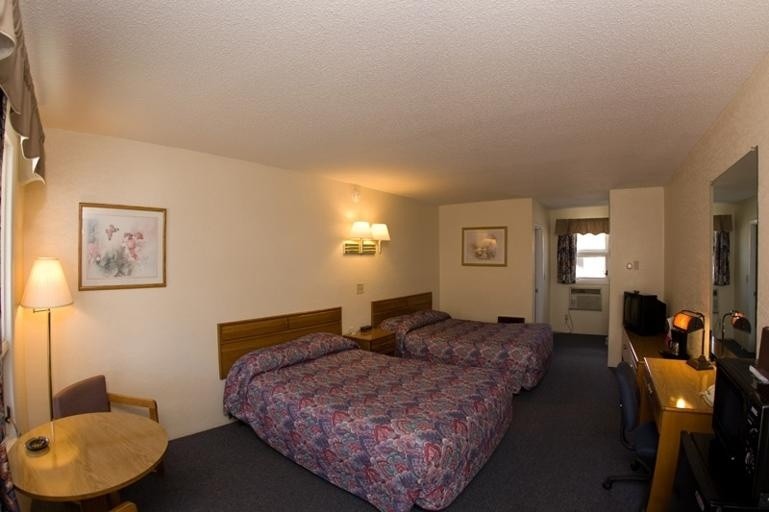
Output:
[[346, 324, 395, 352]]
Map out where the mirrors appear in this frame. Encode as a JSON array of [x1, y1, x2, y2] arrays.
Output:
[[707, 145, 758, 366]]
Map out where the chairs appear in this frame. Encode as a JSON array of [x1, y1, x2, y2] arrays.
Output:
[[53, 376, 159, 422]]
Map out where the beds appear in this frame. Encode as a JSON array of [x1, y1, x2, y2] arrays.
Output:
[[371, 292, 552, 393], [217, 308, 515, 512]]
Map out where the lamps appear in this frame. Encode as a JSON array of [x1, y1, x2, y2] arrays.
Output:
[[371, 223, 392, 253], [351, 221, 373, 253], [19, 258, 73, 422], [672, 309, 711, 369], [718, 310, 749, 353]]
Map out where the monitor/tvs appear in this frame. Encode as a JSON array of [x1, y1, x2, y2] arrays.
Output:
[[623, 290, 666, 336]]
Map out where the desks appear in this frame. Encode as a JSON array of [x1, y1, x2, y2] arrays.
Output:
[[9, 410, 169, 501], [636, 357, 716, 512]]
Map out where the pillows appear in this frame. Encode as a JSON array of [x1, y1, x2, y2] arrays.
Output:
[[243, 331, 355, 374], [384, 308, 450, 333]]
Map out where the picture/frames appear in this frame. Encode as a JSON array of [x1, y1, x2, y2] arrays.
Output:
[[461, 227, 507, 266], [76, 203, 168, 292]]
